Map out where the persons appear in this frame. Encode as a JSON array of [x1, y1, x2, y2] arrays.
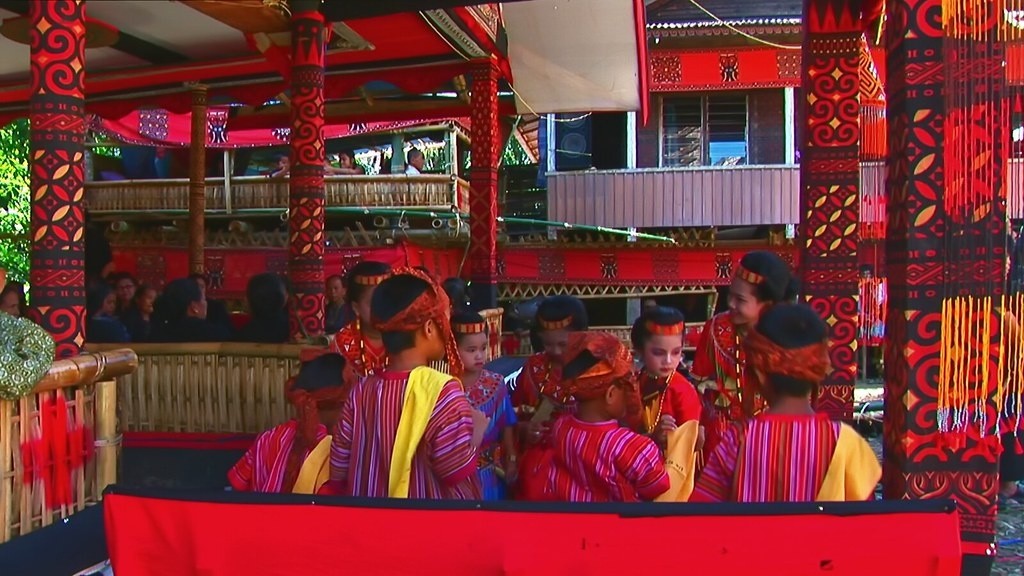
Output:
[[227, 249, 882, 503], [86, 262, 468, 341], [0, 281, 26, 318], [271, 149, 425, 179]]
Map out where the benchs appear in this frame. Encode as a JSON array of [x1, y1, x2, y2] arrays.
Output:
[[101, 483, 962, 576]]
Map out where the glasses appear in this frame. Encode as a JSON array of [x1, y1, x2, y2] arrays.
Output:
[[117, 285, 135, 290]]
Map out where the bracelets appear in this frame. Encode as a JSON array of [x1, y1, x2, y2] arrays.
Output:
[[508, 455, 516, 462]]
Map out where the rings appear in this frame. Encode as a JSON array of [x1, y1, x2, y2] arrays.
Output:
[[536, 432, 539, 435]]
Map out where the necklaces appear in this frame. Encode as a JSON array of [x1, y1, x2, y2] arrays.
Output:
[[735, 329, 768, 416], [637, 365, 675, 433], [356, 320, 389, 375]]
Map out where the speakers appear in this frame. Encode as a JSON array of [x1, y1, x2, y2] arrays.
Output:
[[555, 113, 594, 170]]
